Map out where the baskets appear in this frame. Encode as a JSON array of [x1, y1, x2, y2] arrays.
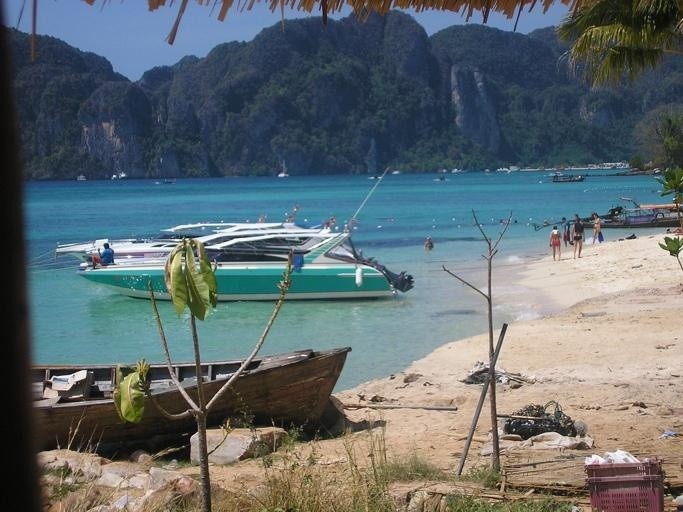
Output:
[[585, 457, 666, 512]]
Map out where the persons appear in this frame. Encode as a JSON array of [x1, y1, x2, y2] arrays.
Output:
[[592, 214, 601, 245], [575, 215, 585, 241], [425, 236, 433, 251], [571, 218, 585, 259], [91, 243, 116, 269], [549, 226, 562, 261], [562, 218, 573, 247]]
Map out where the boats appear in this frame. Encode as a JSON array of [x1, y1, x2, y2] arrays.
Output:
[[440, 162, 631, 174], [153, 179, 177, 185], [31, 345, 353, 451], [531, 196, 682, 230], [76, 175, 87, 183], [432, 178, 449, 182], [548, 172, 563, 177], [552, 175, 585, 183], [111, 172, 129, 181], [392, 170, 400, 175], [368, 176, 383, 181], [276, 160, 289, 178]]
